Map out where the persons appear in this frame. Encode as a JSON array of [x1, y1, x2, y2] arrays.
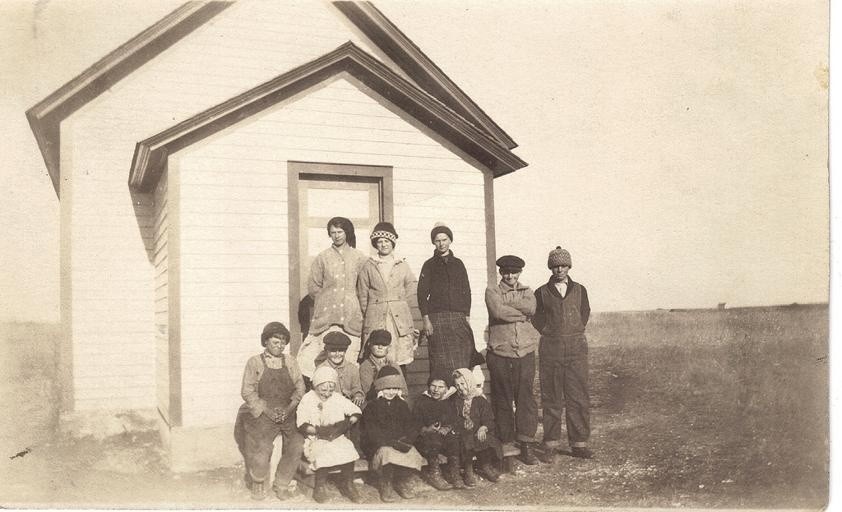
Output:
[[357, 222, 421, 363], [299, 216, 371, 384], [361, 366, 421, 499], [314, 331, 365, 457], [452, 368, 501, 487], [416, 223, 476, 378], [482, 256, 541, 471], [362, 329, 409, 402], [411, 369, 462, 491], [234, 320, 306, 501], [531, 246, 595, 464], [297, 364, 364, 504]]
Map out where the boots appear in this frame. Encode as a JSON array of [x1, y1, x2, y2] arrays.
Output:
[[340, 472, 362, 502], [313, 473, 327, 503], [395, 469, 416, 498], [447, 456, 463, 488], [427, 463, 452, 490], [379, 467, 396, 502]]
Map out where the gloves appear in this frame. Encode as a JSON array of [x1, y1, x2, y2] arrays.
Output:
[[315, 424, 338, 440], [473, 426, 487, 442], [390, 441, 410, 452], [329, 419, 351, 439]]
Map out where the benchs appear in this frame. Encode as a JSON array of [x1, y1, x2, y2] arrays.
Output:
[[297, 445, 521, 501]]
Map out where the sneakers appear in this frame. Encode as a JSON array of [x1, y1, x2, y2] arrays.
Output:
[[543, 449, 559, 462], [463, 471, 475, 487], [273, 486, 291, 501], [520, 444, 537, 465], [251, 482, 265, 499], [570, 447, 596, 458], [477, 464, 497, 481]]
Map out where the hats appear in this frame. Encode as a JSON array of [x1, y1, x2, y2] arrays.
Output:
[[312, 365, 338, 387], [370, 330, 391, 345], [547, 246, 571, 268], [430, 221, 453, 242], [323, 331, 350, 349], [375, 367, 403, 388], [370, 222, 398, 244], [260, 321, 290, 345], [426, 366, 451, 387], [495, 255, 524, 272]]
